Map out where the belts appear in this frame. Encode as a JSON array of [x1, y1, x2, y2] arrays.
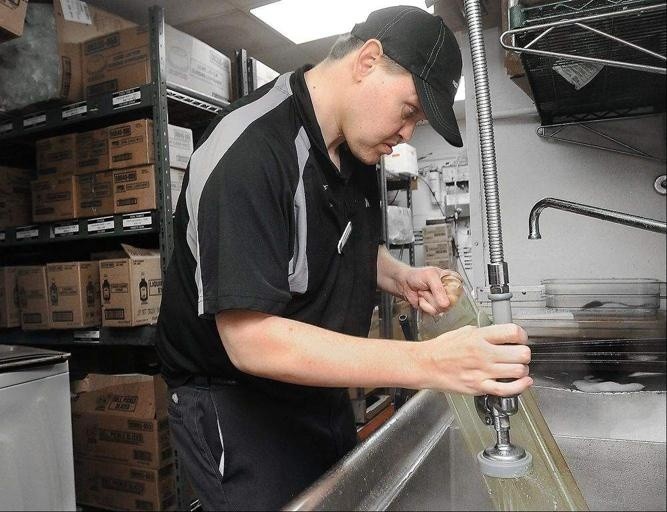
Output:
[[193, 375, 239, 386]]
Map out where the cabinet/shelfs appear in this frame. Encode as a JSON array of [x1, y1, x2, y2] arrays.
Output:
[[360, 289, 394, 426], [154, 8, 248, 277], [250, 57, 364, 434], [385, 147, 418, 407], [1, 2, 184, 511]]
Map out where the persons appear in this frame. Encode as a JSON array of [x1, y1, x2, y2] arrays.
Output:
[[155, 5, 534, 511]]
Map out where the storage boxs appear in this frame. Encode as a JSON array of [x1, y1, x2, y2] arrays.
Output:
[[0, 0, 140, 102], [422, 223, 457, 272]]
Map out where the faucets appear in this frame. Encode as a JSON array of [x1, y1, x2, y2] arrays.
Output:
[[527, 197, 667, 240]]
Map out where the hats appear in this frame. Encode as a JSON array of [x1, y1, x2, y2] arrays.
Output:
[[351, 6, 464, 147]]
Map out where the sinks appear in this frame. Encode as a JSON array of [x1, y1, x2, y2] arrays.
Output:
[[526, 339, 666, 393]]
